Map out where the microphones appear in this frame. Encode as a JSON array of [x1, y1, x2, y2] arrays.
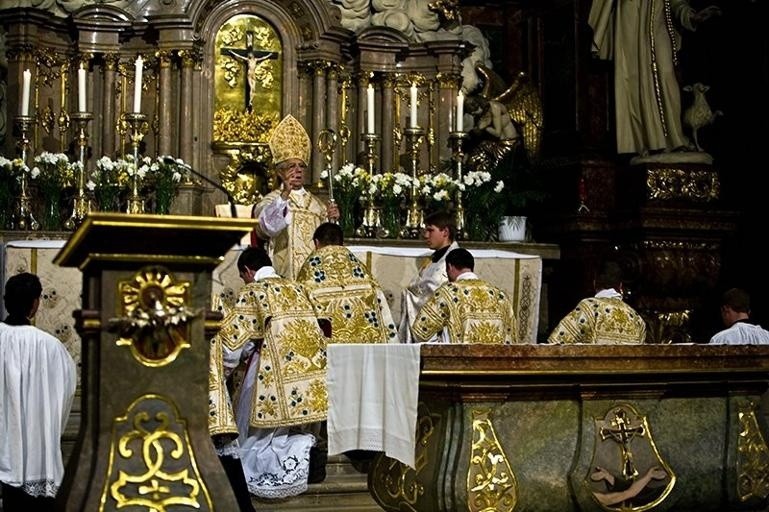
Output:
[[163, 156, 238, 217]]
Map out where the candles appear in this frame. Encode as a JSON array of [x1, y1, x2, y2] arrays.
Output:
[[22, 70, 32, 117], [410, 87, 418, 129], [367, 88, 376, 134], [133, 59, 143, 113], [456, 95, 464, 132], [77, 68, 87, 113]]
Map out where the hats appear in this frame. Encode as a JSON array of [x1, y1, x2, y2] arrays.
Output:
[[269, 115, 313, 167]]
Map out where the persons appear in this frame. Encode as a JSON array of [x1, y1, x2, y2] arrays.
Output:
[[411, 247, 519, 346], [295, 221, 402, 345], [253, 116, 340, 284], [0, 273, 77, 511], [398, 209, 461, 343], [463, 97, 517, 144], [544, 263, 647, 345], [207, 245, 328, 500], [709, 288, 769, 345]]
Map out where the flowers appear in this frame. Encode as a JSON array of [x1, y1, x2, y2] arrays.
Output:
[[0, 153, 193, 203], [321, 165, 504, 222]]
